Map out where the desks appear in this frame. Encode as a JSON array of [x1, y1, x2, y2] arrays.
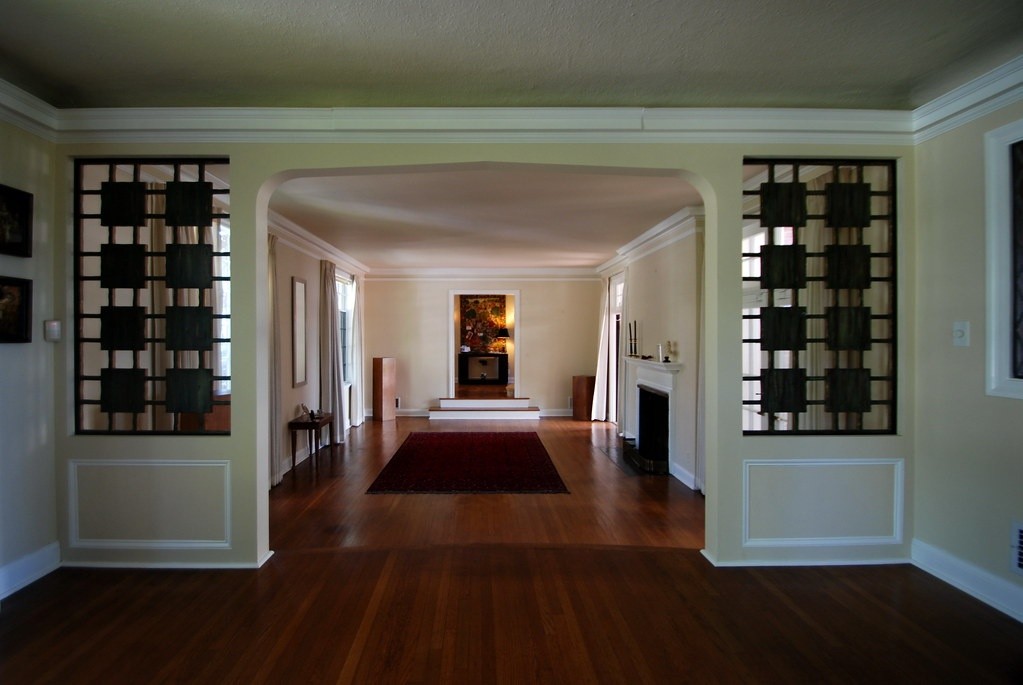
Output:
[[287, 413, 335, 474], [457, 353, 508, 386]]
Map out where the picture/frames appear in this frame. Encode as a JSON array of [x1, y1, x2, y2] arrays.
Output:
[[291, 275, 308, 389]]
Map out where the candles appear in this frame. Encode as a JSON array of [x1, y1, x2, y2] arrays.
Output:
[[633, 319, 637, 339], [629, 322, 632, 339]]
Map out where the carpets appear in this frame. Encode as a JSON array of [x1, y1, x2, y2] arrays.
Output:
[[365, 430, 572, 495]]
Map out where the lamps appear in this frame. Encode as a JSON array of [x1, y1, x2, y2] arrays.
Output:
[[496, 329, 510, 353]]
